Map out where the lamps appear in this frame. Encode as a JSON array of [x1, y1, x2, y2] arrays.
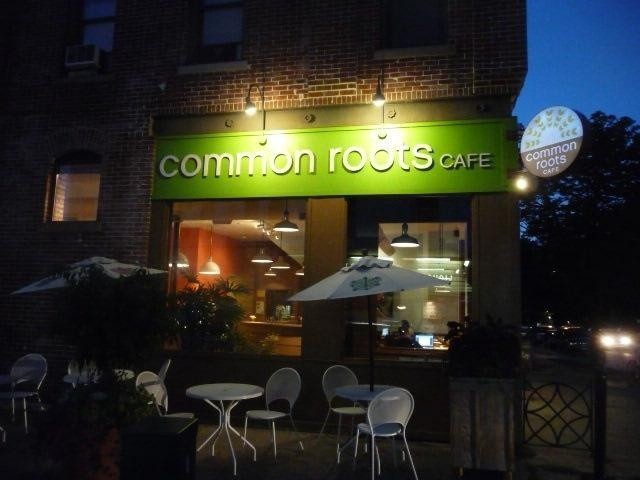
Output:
[[244, 83, 262, 119], [295, 265, 304, 276], [272, 199, 299, 232], [251, 248, 272, 263], [263, 269, 277, 276], [270, 233, 290, 269], [178, 252, 189, 268], [372, 76, 385, 103], [198, 220, 221, 275], [391, 222, 420, 248]]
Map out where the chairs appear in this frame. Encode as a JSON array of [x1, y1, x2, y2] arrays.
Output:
[[239, 367, 304, 465], [351, 387, 419, 480], [0, 354, 48, 434], [316, 365, 367, 464], [134, 371, 194, 473], [158, 358, 171, 380]]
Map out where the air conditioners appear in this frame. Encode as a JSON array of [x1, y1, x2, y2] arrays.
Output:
[[63, 44, 110, 73]]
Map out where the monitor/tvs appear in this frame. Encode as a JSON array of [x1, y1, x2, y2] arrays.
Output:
[[415, 334, 433, 348]]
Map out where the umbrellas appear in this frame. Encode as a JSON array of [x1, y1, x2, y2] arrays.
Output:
[[287, 247, 453, 393], [6, 256, 171, 297]]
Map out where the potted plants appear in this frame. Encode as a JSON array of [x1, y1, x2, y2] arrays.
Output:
[[445, 315, 523, 479]]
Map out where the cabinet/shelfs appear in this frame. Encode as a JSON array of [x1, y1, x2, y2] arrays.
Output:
[[122, 416, 200, 480]]
